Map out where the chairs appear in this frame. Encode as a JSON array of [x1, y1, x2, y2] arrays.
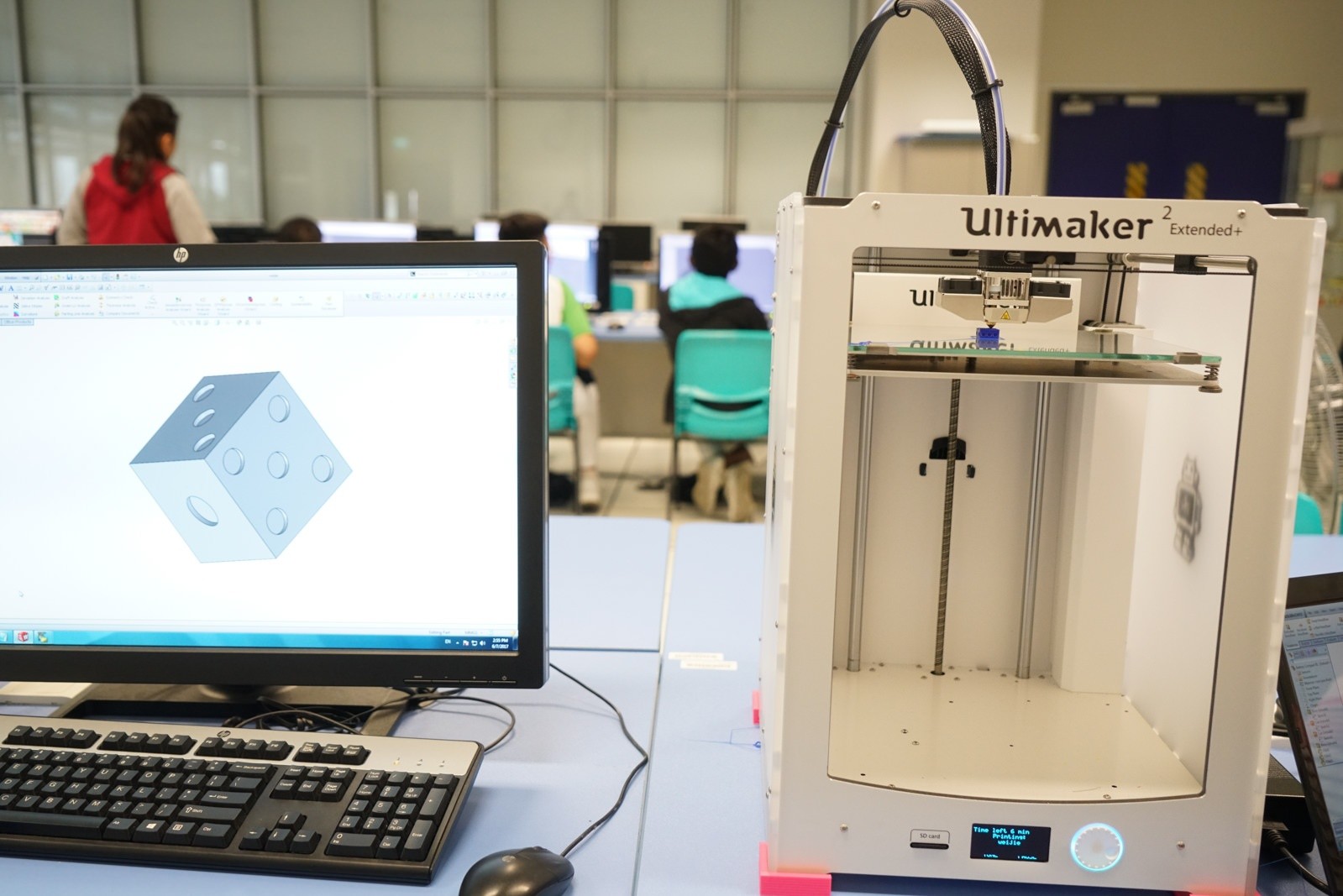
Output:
[[673, 330, 772, 521], [545, 328, 602, 510], [612, 283, 632, 312]]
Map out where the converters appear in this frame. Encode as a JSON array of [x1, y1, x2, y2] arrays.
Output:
[[1257, 753, 1315, 855]]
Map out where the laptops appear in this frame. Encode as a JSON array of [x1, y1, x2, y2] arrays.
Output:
[[1277, 570, 1343, 896]]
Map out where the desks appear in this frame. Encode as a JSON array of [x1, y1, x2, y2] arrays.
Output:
[[0, 517, 1343, 896], [582, 310, 674, 439]]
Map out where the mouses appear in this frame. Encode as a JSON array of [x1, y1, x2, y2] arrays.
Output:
[[459, 845, 574, 896]]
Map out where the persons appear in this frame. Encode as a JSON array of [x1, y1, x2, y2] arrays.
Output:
[[656, 226, 771, 524], [275, 217, 323, 243], [55, 93, 219, 246], [496, 212, 602, 514]]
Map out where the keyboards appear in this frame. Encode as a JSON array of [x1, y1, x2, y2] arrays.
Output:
[[1, 715, 487, 888]]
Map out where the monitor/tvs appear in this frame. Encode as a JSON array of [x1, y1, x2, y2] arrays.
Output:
[[0, 208, 781, 738]]
